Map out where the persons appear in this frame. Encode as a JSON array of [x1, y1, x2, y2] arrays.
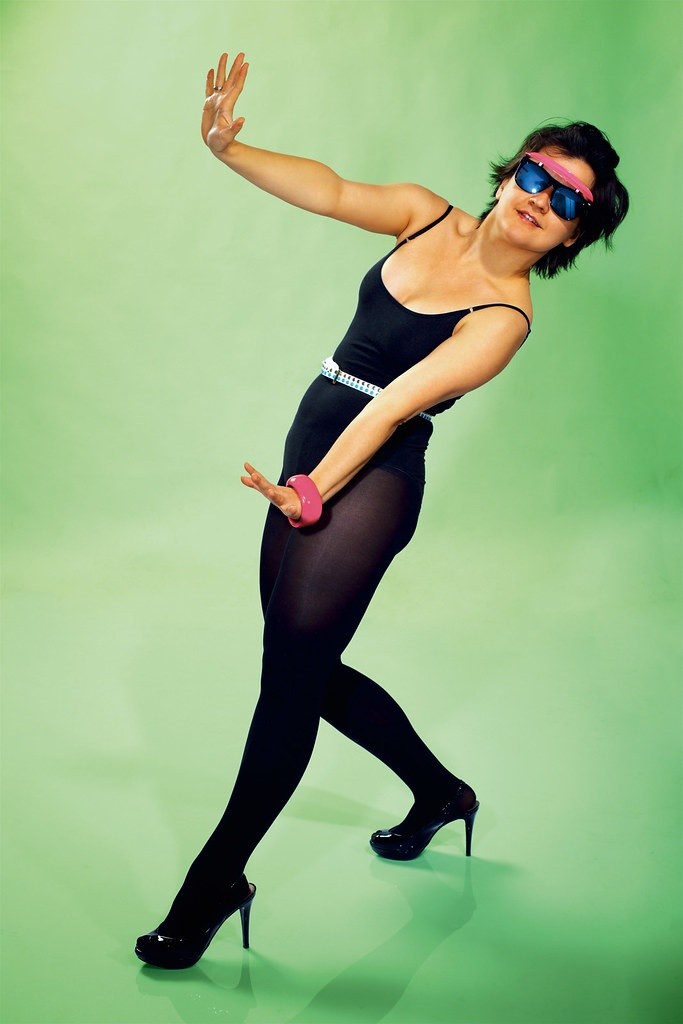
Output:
[[136, 52, 631, 969]]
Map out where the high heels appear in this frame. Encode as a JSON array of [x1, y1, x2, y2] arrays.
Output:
[[369, 780, 480, 860], [135, 874, 257, 971]]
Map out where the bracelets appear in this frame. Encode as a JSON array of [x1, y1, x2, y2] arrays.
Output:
[[286, 475, 323, 528]]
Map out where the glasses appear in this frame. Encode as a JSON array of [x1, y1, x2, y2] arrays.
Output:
[[505, 154, 596, 222]]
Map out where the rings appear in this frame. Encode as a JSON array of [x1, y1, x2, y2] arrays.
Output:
[[213, 85, 222, 90]]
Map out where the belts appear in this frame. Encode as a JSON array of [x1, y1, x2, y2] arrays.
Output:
[[316, 363, 439, 424]]
